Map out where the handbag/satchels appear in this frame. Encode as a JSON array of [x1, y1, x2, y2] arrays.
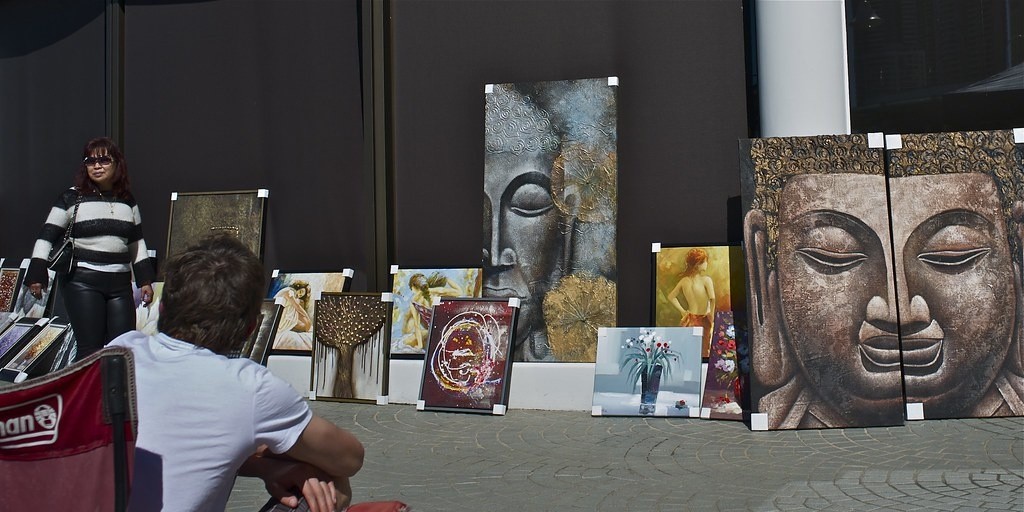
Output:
[[46, 235, 75, 274]]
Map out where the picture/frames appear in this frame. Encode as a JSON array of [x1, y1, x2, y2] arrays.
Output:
[[0, 310, 18, 335], [416, 295, 522, 416], [0, 315, 51, 369], [589, 325, 705, 416], [650, 240, 748, 364], [0, 315, 72, 383], [265, 266, 355, 357], [164, 187, 269, 261], [307, 289, 394, 406], [1, 266, 25, 313], [390, 264, 486, 361]]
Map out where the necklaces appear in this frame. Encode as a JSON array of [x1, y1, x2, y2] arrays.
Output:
[[93, 187, 118, 216]]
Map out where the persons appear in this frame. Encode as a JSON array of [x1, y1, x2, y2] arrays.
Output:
[[24, 136, 156, 363], [104, 232, 367, 512]]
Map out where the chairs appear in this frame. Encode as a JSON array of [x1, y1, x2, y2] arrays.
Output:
[[0, 345, 408, 511]]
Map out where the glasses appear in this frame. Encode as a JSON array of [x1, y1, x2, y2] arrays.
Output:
[[82, 155, 114, 166]]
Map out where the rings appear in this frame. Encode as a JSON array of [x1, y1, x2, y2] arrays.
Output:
[[30, 291, 36, 296]]
